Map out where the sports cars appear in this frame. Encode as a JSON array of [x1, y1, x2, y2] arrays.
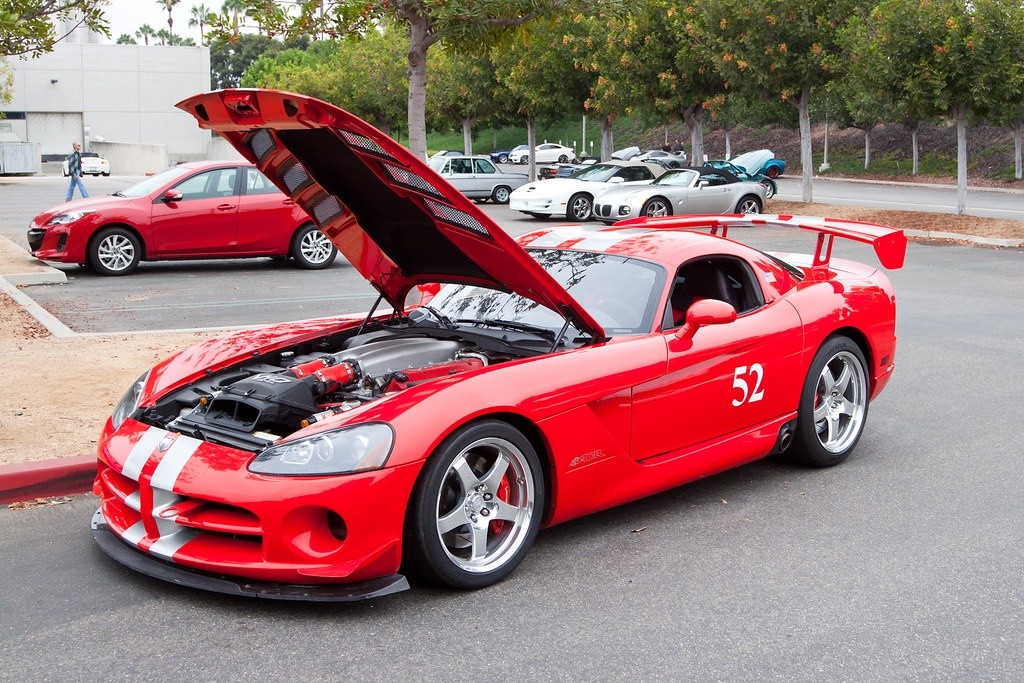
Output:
[[508, 158, 669, 222], [591, 165, 768, 226], [28, 159, 340, 277], [62, 151, 111, 176], [536, 156, 603, 183], [89, 85, 908, 609], [611, 143, 687, 172]]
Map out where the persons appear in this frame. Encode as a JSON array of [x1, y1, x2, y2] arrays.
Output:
[[663, 141, 671, 153], [672, 139, 684, 152], [65, 141, 89, 201]]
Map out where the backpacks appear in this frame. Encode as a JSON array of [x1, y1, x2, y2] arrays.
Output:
[[61, 154, 74, 178]]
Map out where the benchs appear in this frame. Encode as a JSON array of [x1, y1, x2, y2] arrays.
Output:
[[698, 179, 726, 185]]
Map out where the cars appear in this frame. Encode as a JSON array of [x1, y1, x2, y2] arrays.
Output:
[[487, 144, 528, 165], [427, 149, 467, 169], [701, 149, 788, 200], [428, 154, 531, 205], [508, 143, 577, 164]]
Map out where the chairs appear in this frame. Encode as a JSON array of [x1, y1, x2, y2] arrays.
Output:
[[456, 165, 465, 173], [227, 175, 236, 196], [672, 262, 738, 317], [632, 171, 644, 181]]
